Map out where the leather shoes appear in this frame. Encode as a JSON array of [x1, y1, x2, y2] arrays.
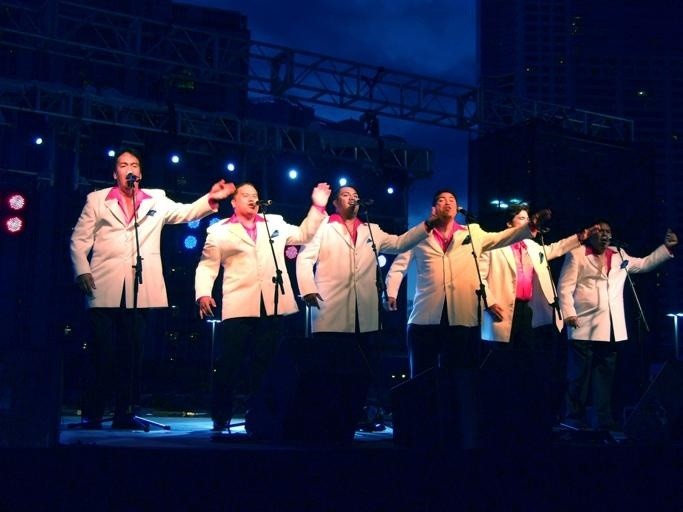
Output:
[[81, 416, 147, 430]]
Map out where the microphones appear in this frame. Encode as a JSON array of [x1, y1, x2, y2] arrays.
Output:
[[458, 206, 480, 221], [354, 196, 368, 209], [607, 236, 630, 248], [253, 199, 272, 207], [536, 227, 550, 232], [126, 171, 137, 182]]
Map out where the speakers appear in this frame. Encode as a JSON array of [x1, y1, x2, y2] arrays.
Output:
[[390, 366, 460, 444], [623, 360, 683, 449]]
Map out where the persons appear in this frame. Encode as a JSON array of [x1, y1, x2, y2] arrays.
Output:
[[295, 186, 438, 443], [384, 190, 552, 377], [68, 152, 237, 429], [479, 208, 600, 434], [556, 223, 678, 432], [194, 182, 331, 432]]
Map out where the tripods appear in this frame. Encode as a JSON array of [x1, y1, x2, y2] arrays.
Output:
[[66, 188, 171, 432], [209, 207, 295, 432]]
